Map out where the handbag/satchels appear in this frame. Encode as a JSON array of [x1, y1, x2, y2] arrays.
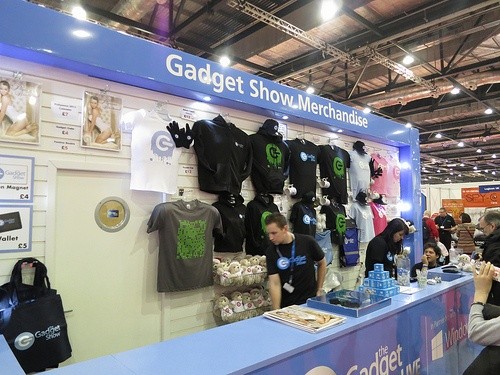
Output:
[[473, 240, 479, 247], [0, 256, 75, 375]]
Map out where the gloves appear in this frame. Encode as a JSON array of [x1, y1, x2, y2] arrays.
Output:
[[182, 122, 196, 148], [166, 120, 183, 147]]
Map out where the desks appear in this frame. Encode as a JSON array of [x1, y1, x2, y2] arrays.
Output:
[[40, 261, 487, 375]]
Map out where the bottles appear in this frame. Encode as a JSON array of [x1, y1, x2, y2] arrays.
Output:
[[475, 261, 500, 283]]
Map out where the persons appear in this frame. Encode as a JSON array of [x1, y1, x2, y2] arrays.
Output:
[[264, 214, 326, 311], [365, 218, 409, 280], [0, 81, 34, 137], [86, 96, 116, 144], [411, 208, 500, 348]]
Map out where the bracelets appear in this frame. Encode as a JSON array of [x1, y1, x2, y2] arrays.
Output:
[[422, 266, 428, 267], [471, 302, 485, 306]]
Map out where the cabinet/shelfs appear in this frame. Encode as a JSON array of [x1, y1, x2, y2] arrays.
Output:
[[212, 270, 273, 327]]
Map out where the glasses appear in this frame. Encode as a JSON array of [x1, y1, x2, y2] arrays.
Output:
[[481, 223, 491, 231]]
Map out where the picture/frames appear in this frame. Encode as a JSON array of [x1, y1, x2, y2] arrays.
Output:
[[0, 74, 41, 145], [81, 90, 122, 152]]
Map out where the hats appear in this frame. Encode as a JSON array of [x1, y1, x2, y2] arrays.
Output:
[[373, 194, 388, 206], [357, 192, 369, 206], [353, 140, 367, 156], [302, 190, 318, 206], [262, 118, 282, 137]]
